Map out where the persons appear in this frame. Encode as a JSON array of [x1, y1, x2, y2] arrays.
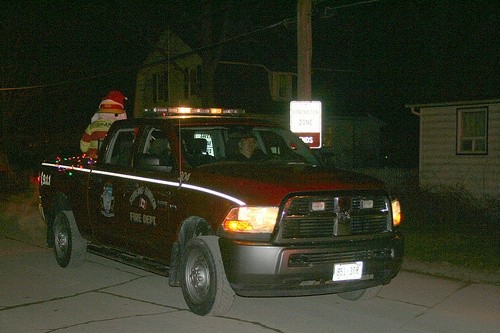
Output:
[[225, 130, 273, 165]]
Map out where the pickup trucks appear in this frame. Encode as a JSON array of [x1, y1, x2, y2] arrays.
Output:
[[36, 106, 408, 321]]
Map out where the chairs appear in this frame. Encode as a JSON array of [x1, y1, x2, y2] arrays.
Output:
[[112, 134, 220, 172]]
